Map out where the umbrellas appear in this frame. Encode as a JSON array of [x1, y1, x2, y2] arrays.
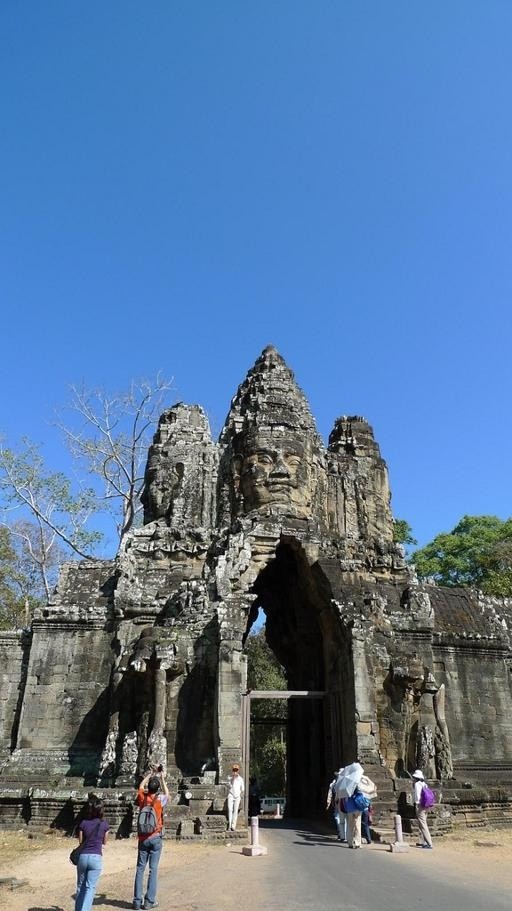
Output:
[[356, 775, 377, 801], [334, 762, 365, 799]]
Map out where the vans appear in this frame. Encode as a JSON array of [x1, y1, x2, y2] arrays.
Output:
[[260, 797, 286, 814]]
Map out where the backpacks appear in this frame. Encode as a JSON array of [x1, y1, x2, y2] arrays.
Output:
[[421, 786, 434, 808], [137, 794, 157, 834]]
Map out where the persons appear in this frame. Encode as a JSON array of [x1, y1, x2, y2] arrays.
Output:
[[326, 768, 373, 850], [411, 770, 433, 850], [227, 428, 324, 517], [226, 766, 244, 832], [75, 796, 110, 911], [134, 765, 169, 910]]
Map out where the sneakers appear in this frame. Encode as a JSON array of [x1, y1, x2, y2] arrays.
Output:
[[134, 899, 159, 910], [416, 843, 432, 848]]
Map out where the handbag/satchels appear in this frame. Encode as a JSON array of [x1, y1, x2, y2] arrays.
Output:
[[231, 764, 239, 770], [70, 844, 83, 865], [340, 788, 370, 813], [412, 770, 424, 780], [148, 776, 160, 793]]
